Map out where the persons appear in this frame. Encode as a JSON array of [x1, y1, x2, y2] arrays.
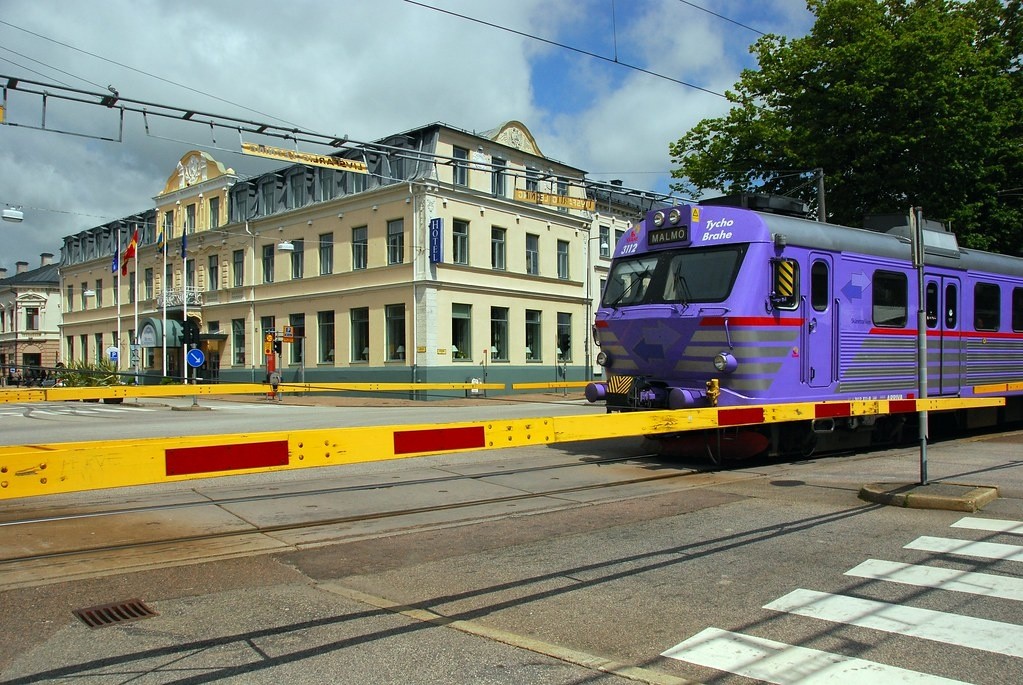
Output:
[[9, 368, 47, 388]]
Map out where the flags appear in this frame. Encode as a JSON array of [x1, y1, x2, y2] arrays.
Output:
[[157, 219, 169, 258], [120, 230, 138, 277], [111, 237, 118, 274]]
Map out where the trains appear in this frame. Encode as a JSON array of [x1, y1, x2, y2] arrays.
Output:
[[581, 205, 1023, 469]]
